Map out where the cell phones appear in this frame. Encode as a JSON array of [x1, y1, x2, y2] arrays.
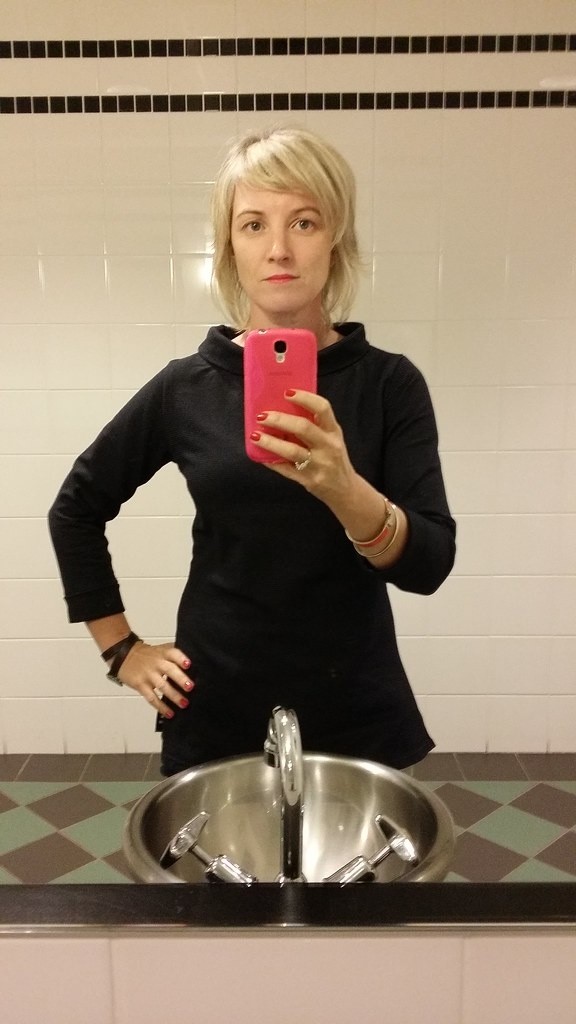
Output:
[[244, 327, 318, 464]]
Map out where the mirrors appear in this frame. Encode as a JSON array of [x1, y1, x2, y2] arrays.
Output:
[[0, 0, 576, 932]]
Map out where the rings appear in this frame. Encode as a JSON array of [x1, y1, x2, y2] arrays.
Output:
[[295, 448, 312, 471]]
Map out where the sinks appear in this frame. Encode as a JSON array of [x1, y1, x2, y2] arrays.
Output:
[[123, 750, 456, 882]]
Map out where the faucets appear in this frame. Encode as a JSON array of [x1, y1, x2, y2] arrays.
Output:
[[262, 701, 307, 883]]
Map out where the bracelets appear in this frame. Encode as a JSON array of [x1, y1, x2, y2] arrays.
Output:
[[106, 631, 139, 687], [98, 636, 144, 663], [353, 500, 400, 559], [345, 492, 394, 547]]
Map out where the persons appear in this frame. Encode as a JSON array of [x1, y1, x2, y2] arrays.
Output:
[[47, 126, 459, 781]]
